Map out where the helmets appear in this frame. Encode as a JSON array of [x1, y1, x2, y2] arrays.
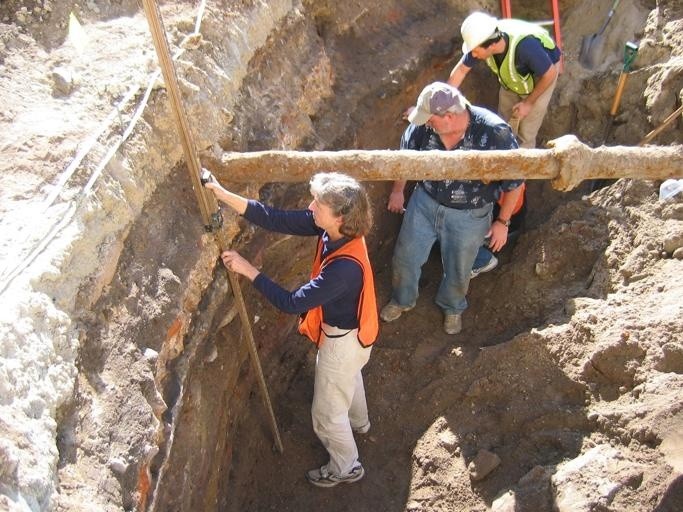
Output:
[[461, 12, 498, 54]]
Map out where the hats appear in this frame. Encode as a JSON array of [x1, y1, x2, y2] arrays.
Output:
[[408, 82, 459, 126]]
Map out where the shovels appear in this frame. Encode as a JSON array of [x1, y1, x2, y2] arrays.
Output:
[[580, 1, 638, 195]]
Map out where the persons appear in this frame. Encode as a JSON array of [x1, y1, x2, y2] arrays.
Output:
[[441, 10, 564, 172], [200, 165, 382, 490], [366, 81, 527, 337], [469, 180, 533, 282]]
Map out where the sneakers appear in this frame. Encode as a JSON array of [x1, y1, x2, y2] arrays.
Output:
[[306, 461, 365, 488], [352, 421, 371, 433], [444, 314, 462, 335], [471, 255, 498, 279], [380, 300, 416, 322]]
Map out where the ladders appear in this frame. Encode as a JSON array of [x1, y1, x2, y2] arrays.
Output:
[[500, 0, 564, 77]]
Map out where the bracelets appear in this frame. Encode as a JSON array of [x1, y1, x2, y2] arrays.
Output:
[[494, 217, 509, 229]]
